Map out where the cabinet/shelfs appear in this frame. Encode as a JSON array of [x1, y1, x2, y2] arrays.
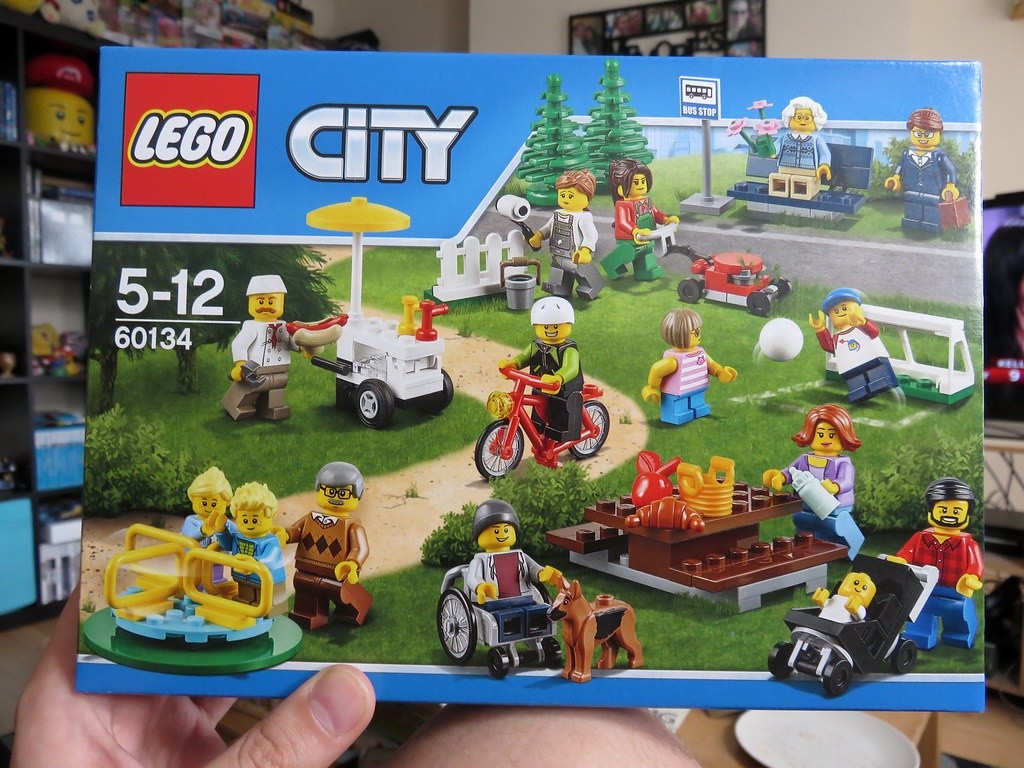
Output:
[[0, 8, 128, 633]]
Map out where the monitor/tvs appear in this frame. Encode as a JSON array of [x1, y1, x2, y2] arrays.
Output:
[[980, 191, 1024, 420]]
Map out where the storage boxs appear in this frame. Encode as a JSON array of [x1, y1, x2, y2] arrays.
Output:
[[0, 0, 986, 714]]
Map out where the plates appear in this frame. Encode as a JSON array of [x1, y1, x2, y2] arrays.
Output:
[[734, 709, 922, 768]]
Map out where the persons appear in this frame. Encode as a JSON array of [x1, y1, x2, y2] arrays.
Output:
[[14, 582, 700, 768], [572, 0, 764, 57]]
